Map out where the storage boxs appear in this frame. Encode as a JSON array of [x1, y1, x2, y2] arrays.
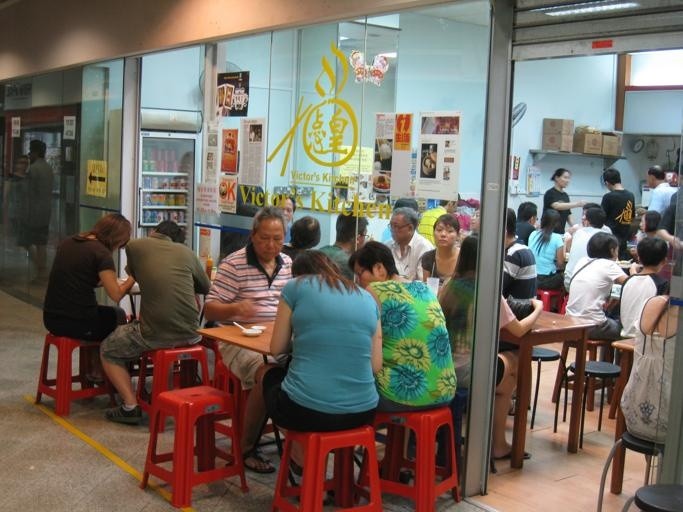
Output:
[[543, 118, 624, 156]]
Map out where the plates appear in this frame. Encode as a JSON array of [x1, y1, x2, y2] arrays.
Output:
[[242, 325, 268, 337]]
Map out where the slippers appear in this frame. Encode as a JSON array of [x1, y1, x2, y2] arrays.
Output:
[[86, 375, 106, 386], [243, 448, 276, 473], [495, 447, 531, 460], [279, 439, 303, 477]]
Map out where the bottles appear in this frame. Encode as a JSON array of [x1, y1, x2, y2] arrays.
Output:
[[138, 146, 186, 225], [205, 259, 212, 282]]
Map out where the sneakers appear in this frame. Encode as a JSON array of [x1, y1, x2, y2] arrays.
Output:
[[105, 403, 143, 423]]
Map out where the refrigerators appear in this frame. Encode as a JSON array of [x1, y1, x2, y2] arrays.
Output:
[[104, 108, 203, 255]]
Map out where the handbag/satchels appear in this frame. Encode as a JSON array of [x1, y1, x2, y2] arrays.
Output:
[[506, 299, 533, 321]]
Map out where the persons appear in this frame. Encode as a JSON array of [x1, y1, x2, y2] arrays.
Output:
[[97, 220, 214, 423], [42, 212, 134, 352], [153, 151, 196, 217], [203, 205, 289, 474], [262, 164, 681, 462], [244, 247, 384, 505], [1, 156, 29, 237], [21, 139, 55, 286], [347, 239, 458, 499]]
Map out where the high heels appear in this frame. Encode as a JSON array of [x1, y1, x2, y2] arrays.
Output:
[[400, 470, 411, 485]]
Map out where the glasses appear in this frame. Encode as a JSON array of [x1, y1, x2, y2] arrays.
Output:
[[357, 268, 365, 278], [361, 234, 369, 239], [388, 223, 409, 231]]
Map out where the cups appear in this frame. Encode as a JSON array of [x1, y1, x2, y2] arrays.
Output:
[[426, 276, 439, 298]]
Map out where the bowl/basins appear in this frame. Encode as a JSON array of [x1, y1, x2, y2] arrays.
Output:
[[421, 156, 436, 177], [372, 173, 391, 193]]
